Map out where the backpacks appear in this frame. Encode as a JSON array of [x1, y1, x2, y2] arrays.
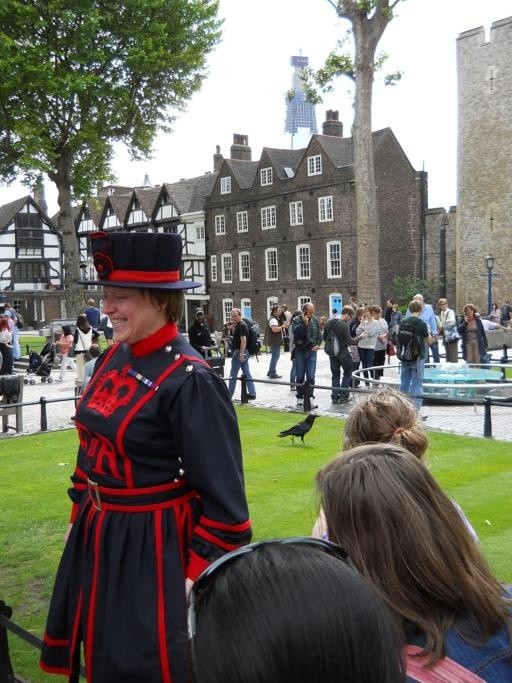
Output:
[[242, 319, 262, 362], [396, 320, 421, 363], [16, 311, 25, 328], [324, 320, 360, 362]]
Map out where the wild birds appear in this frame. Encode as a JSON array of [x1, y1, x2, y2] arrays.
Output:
[[278, 413, 320, 446]]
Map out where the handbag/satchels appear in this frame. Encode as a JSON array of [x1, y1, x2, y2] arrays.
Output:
[[443, 323, 461, 344], [482, 354, 492, 368], [85, 349, 94, 361], [386, 341, 396, 356], [67, 347, 75, 358]]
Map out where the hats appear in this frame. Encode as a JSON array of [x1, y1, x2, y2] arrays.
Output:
[[76, 232, 201, 289], [194, 308, 204, 314]]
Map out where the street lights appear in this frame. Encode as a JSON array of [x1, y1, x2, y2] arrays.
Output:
[[480, 254, 500, 317]]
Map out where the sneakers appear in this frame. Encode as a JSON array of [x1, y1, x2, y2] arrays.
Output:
[[332, 396, 354, 403], [291, 386, 318, 408]]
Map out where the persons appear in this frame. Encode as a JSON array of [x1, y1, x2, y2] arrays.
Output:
[[0, 298, 114, 419], [188, 293, 488, 418], [487, 300, 512, 327], [313, 442, 512, 683], [311, 386, 477, 548], [38, 231, 253, 682], [187, 536, 408, 683]]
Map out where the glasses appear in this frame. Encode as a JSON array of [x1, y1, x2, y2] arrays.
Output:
[[186, 535, 357, 656]]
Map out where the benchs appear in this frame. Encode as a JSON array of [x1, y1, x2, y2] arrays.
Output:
[[215, 331, 268, 356]]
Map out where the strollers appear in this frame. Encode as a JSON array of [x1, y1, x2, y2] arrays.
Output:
[[22, 341, 58, 384]]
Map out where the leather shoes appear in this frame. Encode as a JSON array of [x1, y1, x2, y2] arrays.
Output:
[[247, 394, 256, 399], [267, 372, 281, 378]]
